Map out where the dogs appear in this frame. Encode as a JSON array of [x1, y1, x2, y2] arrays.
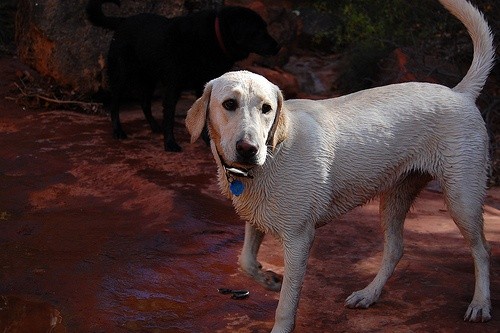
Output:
[[185, 0, 497, 333], [85, 0, 281, 154]]
